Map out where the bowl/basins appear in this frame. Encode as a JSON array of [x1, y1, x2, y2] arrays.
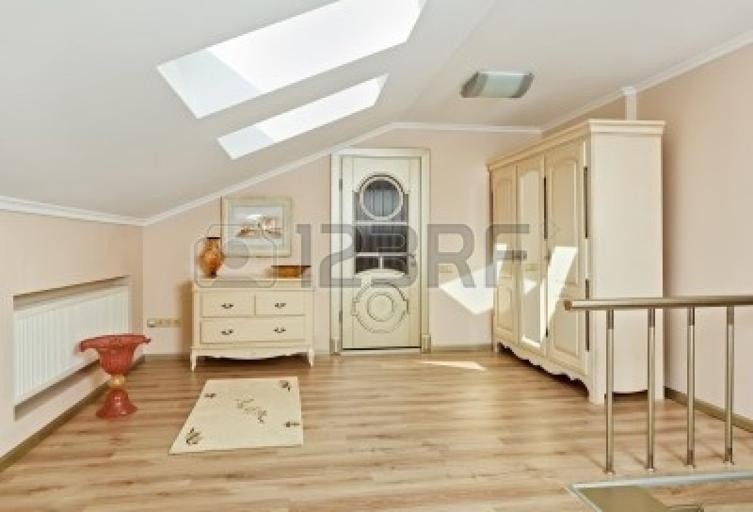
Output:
[[272, 265, 311, 278]]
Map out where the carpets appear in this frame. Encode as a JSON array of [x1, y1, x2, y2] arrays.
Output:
[[167, 376, 305, 454]]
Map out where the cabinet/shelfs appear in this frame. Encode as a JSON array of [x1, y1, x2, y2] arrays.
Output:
[[190, 278, 318, 369], [491, 119, 665, 406]]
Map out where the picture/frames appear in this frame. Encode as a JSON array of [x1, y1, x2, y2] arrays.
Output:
[[220, 194, 294, 259]]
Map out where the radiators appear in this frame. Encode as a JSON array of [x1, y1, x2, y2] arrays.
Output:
[[14, 281, 134, 411]]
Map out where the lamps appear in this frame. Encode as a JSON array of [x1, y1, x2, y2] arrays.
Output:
[[461, 69, 534, 99]]
